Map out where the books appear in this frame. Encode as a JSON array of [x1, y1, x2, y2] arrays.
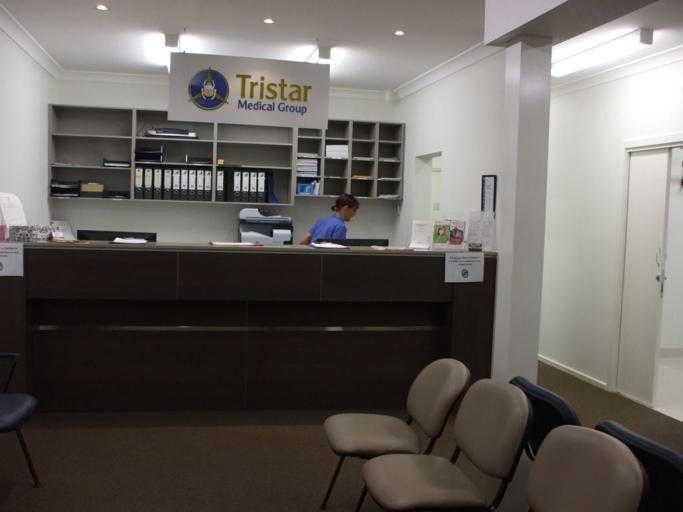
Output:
[[296, 157, 319, 176], [104, 160, 131, 167], [188, 157, 211, 163]]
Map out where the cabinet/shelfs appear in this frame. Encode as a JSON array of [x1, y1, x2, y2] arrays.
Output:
[[47, 104, 132, 201], [134, 109, 214, 204], [214, 122, 295, 203], [296, 119, 405, 198]]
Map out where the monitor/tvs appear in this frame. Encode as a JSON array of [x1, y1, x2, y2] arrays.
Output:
[[317, 239, 388, 246], [77, 230, 156, 242]]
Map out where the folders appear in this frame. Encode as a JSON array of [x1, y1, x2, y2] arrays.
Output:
[[134, 167, 211, 201], [224, 170, 266, 203], [216, 170, 224, 202]]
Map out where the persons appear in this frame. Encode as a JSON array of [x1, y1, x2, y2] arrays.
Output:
[[436, 227, 447, 243], [299, 194, 360, 245]]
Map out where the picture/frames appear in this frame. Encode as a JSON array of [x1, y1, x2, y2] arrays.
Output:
[[481, 175, 496, 211]]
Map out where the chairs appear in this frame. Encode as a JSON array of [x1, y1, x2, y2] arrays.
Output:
[[0, 352, 41, 488], [353, 379, 533, 512], [595, 419, 683, 512], [510, 376, 580, 457], [524, 424, 644, 512], [321, 357, 472, 509]]
